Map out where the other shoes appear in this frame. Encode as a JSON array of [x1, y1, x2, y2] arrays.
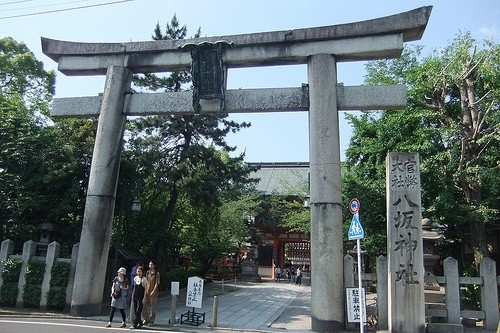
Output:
[[137, 321, 143, 327], [105, 324, 112, 327], [142, 320, 148, 324], [120, 323, 126, 328], [130, 325, 138, 329], [148, 321, 154, 327]]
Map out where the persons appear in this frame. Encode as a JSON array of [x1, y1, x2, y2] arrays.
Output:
[[105, 257, 160, 329], [274, 266, 302, 287]]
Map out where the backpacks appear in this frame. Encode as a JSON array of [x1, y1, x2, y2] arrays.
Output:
[[112, 276, 128, 299]]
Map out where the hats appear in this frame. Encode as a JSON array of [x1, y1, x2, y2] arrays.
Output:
[[117, 267, 126, 275]]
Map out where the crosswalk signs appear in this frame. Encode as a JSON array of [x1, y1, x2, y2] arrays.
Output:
[[346, 214, 367, 331]]
